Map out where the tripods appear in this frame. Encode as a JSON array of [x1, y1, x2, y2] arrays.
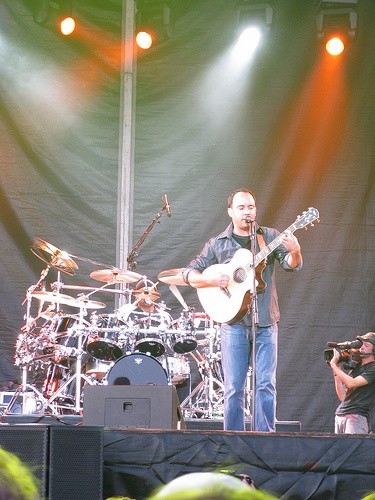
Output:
[[2, 264, 119, 417], [180, 319, 252, 421]]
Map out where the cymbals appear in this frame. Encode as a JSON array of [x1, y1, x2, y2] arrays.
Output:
[[89, 268, 144, 284], [30, 290, 107, 310], [33, 236, 79, 269], [157, 267, 189, 286], [38, 311, 66, 320], [128, 289, 161, 301]]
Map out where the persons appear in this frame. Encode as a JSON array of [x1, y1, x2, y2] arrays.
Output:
[[329, 332, 375, 434], [118, 279, 174, 332], [182, 188, 303, 432], [0, 448, 375, 500]]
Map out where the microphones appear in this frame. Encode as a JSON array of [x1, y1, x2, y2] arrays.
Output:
[[357, 351, 375, 355], [163, 195, 172, 217], [246, 216, 256, 223], [327, 342, 338, 347]]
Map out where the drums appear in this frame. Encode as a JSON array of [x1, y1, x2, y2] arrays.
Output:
[[85, 314, 132, 361], [42, 316, 92, 369], [132, 316, 169, 358], [104, 351, 168, 386], [55, 368, 88, 404], [83, 356, 114, 377], [166, 319, 199, 355], [160, 355, 191, 385]]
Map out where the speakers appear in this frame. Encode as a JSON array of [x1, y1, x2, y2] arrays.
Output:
[[0, 425, 103, 500], [83, 383, 187, 430]]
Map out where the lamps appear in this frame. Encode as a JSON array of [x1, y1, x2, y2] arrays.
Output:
[[317, 8, 358, 55], [34, 0, 76, 35], [136, 0, 170, 49]]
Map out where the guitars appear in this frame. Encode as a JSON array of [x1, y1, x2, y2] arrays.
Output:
[[196, 206, 321, 326]]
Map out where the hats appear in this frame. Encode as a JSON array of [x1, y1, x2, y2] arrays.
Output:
[[136, 279, 157, 291], [356, 332, 375, 344]]
